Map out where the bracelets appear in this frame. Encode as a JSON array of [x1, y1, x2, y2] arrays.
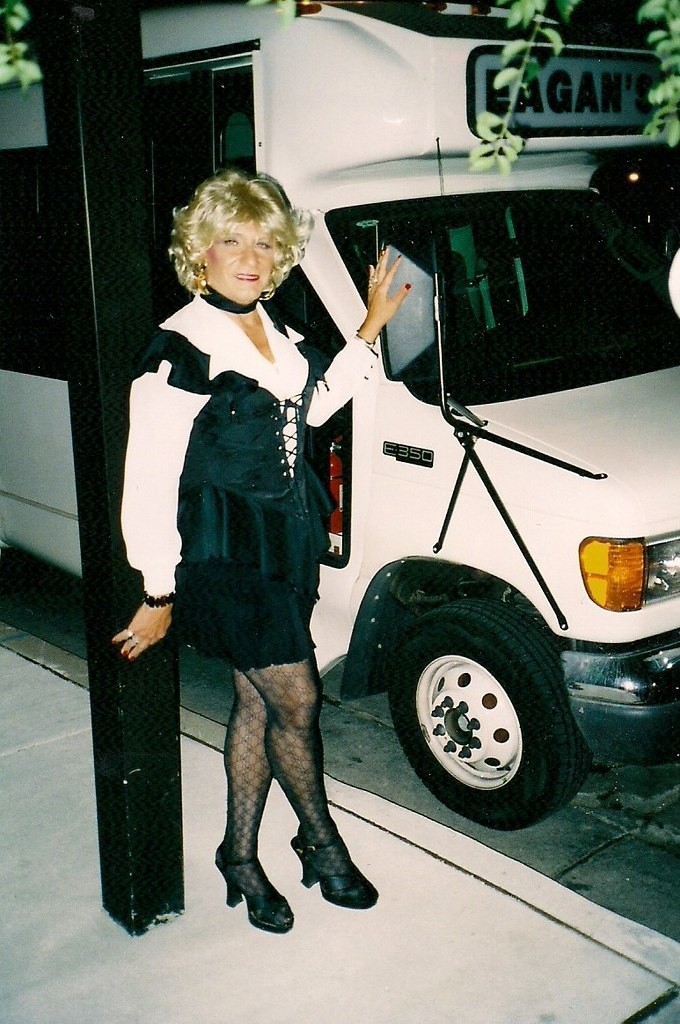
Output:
[[144, 591, 177, 609]]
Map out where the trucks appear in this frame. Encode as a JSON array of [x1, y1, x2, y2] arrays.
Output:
[[0, 0, 680, 830]]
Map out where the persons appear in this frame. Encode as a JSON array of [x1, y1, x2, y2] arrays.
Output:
[[109, 166, 414, 935]]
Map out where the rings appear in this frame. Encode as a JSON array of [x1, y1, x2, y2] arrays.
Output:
[[368, 278, 378, 289], [128, 638, 139, 647], [125, 629, 134, 637]]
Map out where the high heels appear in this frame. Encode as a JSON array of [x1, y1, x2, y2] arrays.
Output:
[[290, 836, 379, 910], [215, 843, 294, 935]]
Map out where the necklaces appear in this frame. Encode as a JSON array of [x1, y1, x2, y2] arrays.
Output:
[[199, 284, 258, 314]]
